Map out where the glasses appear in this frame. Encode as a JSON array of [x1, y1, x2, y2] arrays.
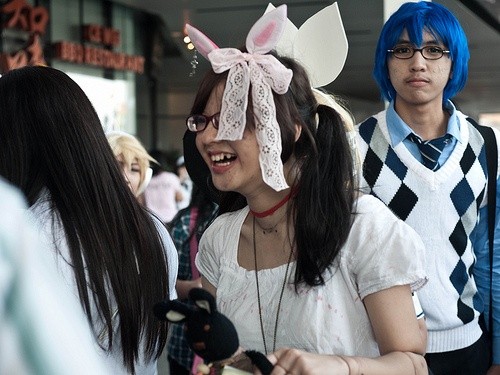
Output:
[[387, 45, 451, 60], [185, 112, 220, 132]]
